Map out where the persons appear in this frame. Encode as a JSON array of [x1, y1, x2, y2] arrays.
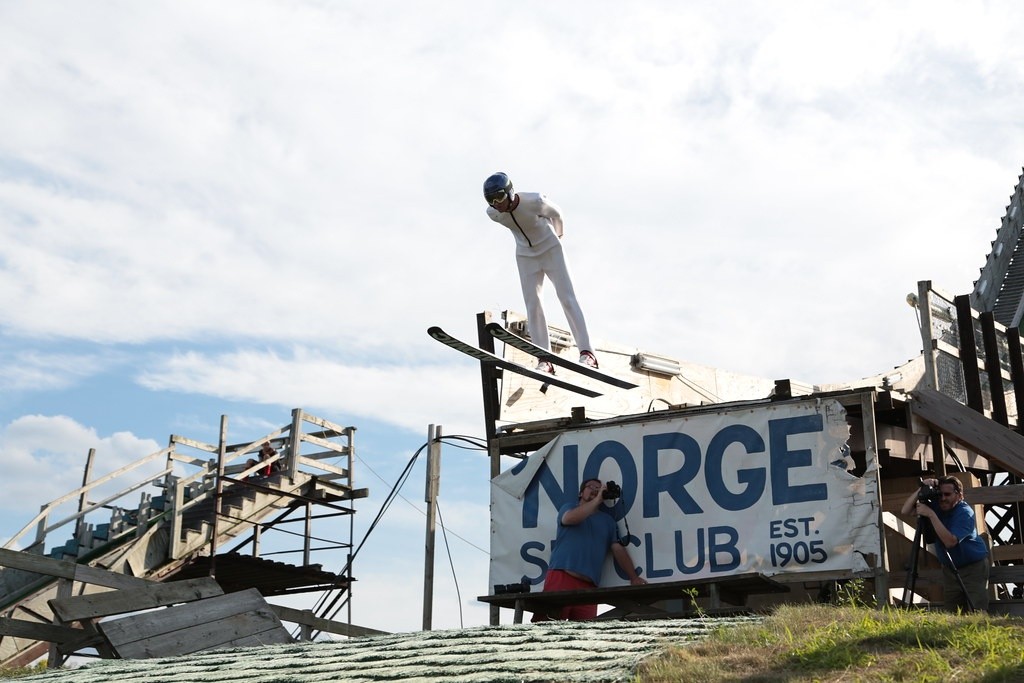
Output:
[[484, 172, 599, 374], [242, 440, 281, 482], [531, 479, 647, 622], [901, 476, 988, 610]]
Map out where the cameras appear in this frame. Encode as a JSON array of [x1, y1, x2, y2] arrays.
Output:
[[602, 481, 620, 499], [918, 484, 936, 502]]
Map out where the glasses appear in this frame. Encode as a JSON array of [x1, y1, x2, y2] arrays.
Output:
[[485, 189, 507, 205], [942, 491, 955, 497], [585, 484, 601, 491]]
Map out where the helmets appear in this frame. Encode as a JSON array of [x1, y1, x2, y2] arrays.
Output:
[[483, 172, 515, 202]]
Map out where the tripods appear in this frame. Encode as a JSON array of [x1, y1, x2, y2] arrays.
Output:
[[901, 499, 977, 615]]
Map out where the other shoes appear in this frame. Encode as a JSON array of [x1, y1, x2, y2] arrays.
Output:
[[534, 361, 553, 375], [578, 351, 597, 368]]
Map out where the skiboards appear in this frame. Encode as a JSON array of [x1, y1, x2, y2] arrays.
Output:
[[425, 322, 639, 397]]
